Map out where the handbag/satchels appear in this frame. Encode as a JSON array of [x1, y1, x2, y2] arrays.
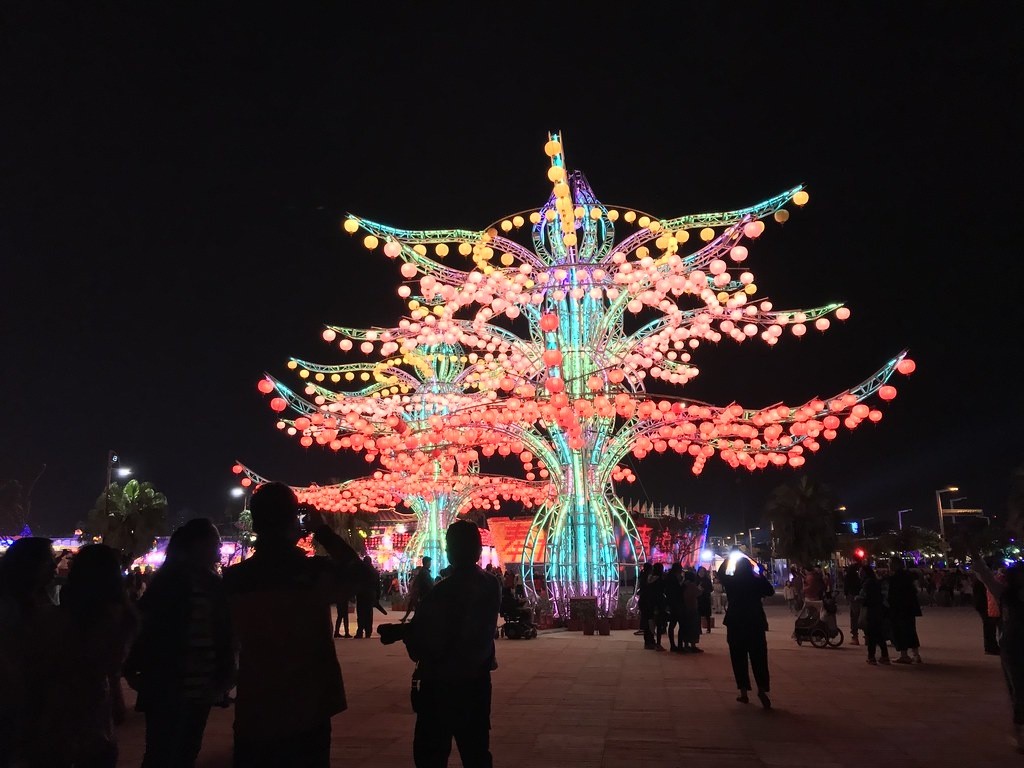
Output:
[[410, 667, 424, 713]]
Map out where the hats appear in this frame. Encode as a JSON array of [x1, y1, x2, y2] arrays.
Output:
[[668, 563, 683, 572]]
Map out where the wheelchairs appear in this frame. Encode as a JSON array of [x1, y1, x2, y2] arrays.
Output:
[[494, 611, 538, 640], [795, 589, 844, 649]]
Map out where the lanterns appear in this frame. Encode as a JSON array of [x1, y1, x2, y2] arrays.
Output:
[[231, 141, 913, 523]]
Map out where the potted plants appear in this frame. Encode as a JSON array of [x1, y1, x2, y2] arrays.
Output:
[[566, 593, 641, 636]]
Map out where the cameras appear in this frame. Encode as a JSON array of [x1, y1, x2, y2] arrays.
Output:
[[377, 622, 412, 645], [296, 510, 308, 525]]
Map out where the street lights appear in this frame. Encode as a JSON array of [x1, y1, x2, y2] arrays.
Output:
[[936, 487, 959, 568], [862, 517, 875, 536], [749, 527, 761, 558], [722, 537, 731, 546], [734, 532, 744, 545], [898, 508, 913, 531]]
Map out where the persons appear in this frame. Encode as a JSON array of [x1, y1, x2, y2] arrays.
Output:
[[0, 482, 547, 768], [637, 546, 1024, 755]]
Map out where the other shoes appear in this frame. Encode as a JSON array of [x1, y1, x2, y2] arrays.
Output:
[[757, 691, 771, 708], [685, 646, 693, 653], [911, 654, 921, 664], [334, 633, 343, 638], [984, 645, 1001, 655], [344, 633, 352, 638], [644, 643, 655, 649], [706, 630, 710, 633], [670, 646, 678, 652], [691, 646, 704, 653], [655, 644, 666, 651], [891, 656, 912, 663], [849, 639, 859, 644], [398, 618, 406, 622], [354, 634, 362, 638], [678, 646, 684, 653], [865, 657, 876, 664], [878, 656, 890, 664], [736, 695, 748, 702]]
